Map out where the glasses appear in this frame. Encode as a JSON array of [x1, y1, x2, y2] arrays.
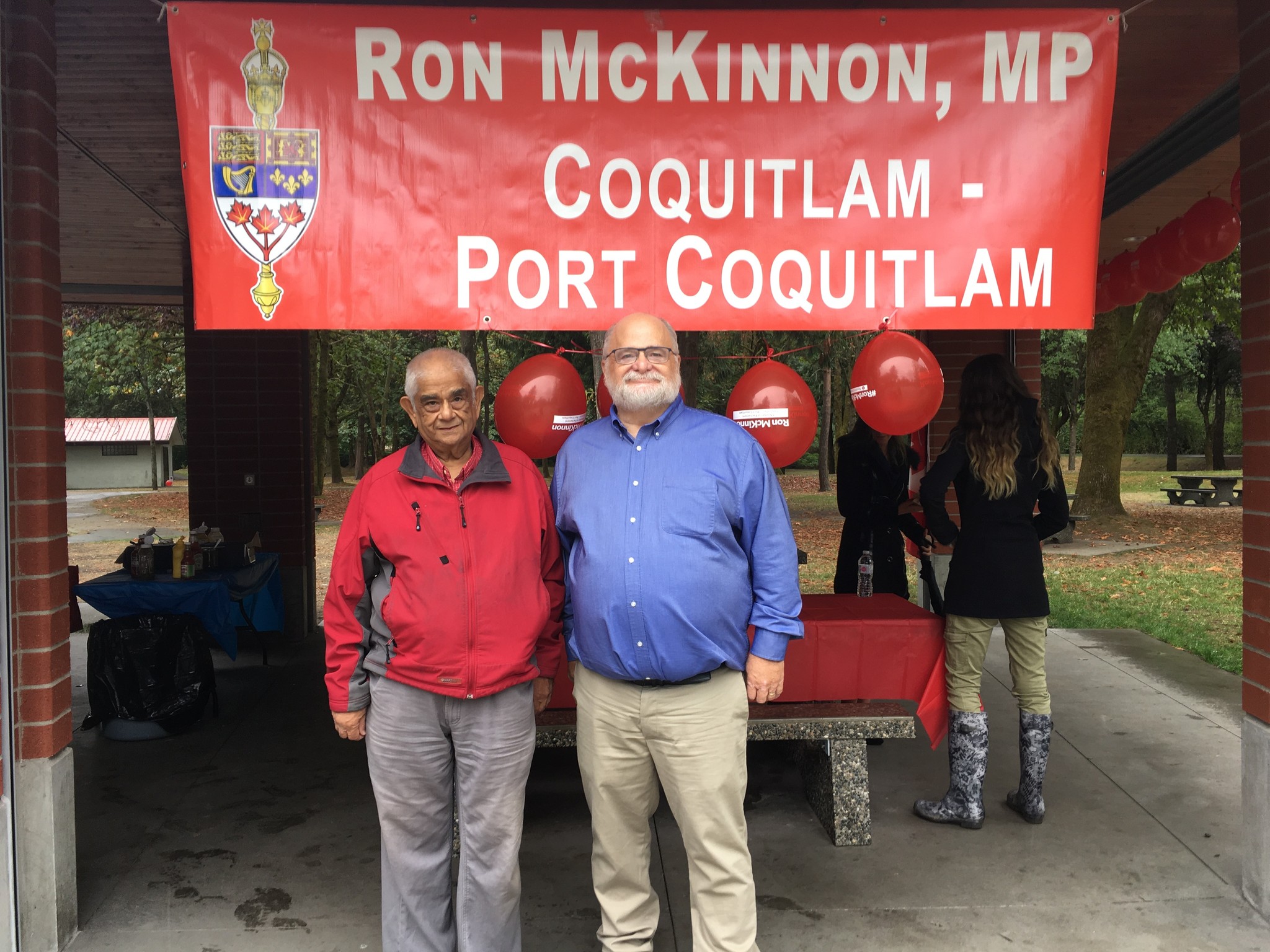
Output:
[[607, 346, 677, 364]]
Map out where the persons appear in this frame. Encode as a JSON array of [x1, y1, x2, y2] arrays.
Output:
[[322, 348, 571, 950], [833, 411, 935, 602], [908, 352, 1072, 830], [547, 314, 809, 952]]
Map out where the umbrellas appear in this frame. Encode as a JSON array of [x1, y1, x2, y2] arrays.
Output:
[[917, 535, 948, 619]]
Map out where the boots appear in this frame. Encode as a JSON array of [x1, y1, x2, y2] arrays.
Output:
[[1006, 708, 1053, 824], [912, 710, 989, 830]]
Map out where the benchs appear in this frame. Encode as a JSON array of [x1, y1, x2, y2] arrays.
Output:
[[1065, 515, 1089, 544], [452, 703, 915, 853], [1199, 488, 1243, 506], [1160, 488, 1218, 507]]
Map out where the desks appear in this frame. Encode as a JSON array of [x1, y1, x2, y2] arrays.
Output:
[[1167, 476, 1243, 508], [71, 550, 287, 716], [545, 592, 985, 751], [1042, 494, 1080, 544]]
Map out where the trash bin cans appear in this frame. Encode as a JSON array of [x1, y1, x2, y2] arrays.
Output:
[[80, 612, 219, 742]]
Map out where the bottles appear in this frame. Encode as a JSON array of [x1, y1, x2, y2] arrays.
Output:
[[857, 550, 874, 597], [131, 528, 156, 577], [172, 522, 225, 581]]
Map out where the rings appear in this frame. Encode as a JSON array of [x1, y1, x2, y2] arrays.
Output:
[[768, 692, 776, 696]]
